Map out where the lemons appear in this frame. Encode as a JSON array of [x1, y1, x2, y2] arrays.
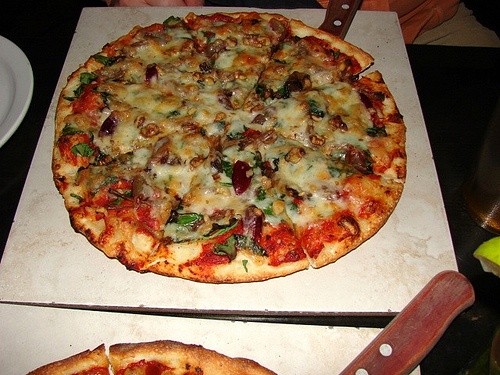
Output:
[[473, 237, 500, 278]]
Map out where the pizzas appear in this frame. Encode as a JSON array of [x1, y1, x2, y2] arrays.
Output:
[[29, 340, 273, 372], [51, 11, 407, 283]]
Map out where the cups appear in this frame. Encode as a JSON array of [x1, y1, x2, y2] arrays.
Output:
[[466, 108, 500, 238]]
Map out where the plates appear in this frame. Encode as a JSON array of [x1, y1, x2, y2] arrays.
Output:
[[0, 33, 36, 147]]
